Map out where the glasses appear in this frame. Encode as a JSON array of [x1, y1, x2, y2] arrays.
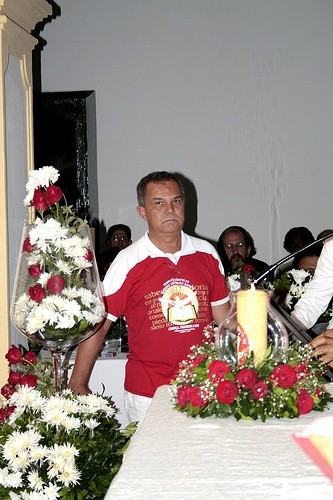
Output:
[[222, 242, 248, 249], [110, 236, 129, 243]]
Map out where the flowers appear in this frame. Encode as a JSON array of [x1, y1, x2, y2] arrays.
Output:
[[168, 260, 333, 422], [3, 166, 138, 500]]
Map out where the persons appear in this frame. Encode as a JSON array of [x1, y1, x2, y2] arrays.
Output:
[[283, 227, 333, 368], [98, 224, 132, 276], [216, 227, 275, 282], [68, 171, 237, 427]]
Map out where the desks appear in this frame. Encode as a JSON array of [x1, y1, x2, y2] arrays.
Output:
[[103, 384, 333, 500], [60, 351, 129, 429]]
